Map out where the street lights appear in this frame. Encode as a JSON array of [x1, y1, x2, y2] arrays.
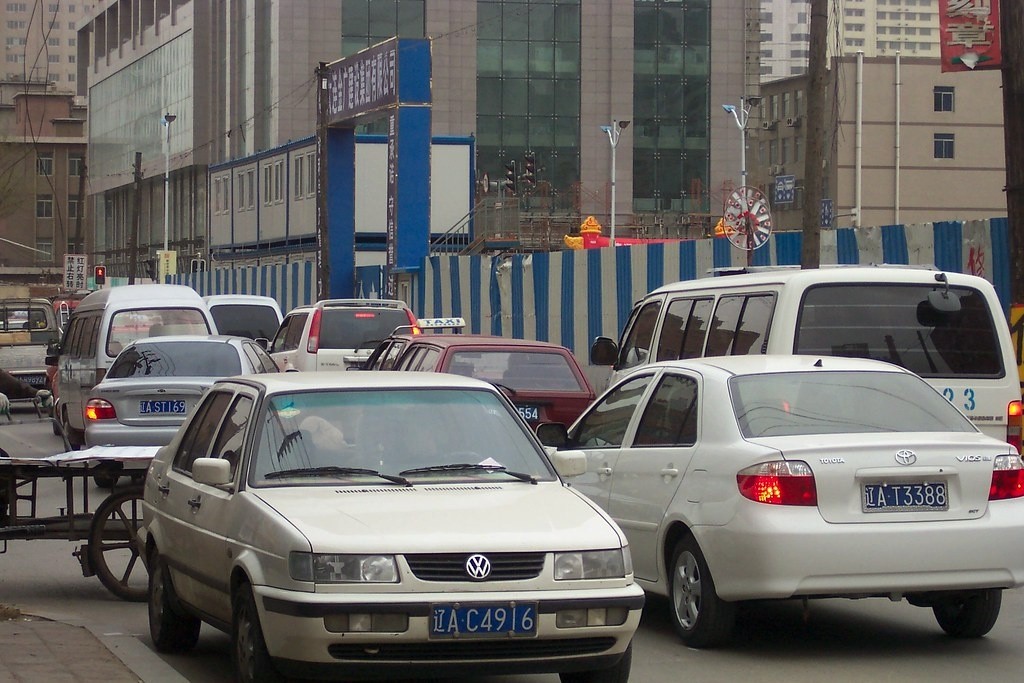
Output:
[[600, 119, 633, 245], [722, 94, 765, 186], [159, 112, 179, 251]]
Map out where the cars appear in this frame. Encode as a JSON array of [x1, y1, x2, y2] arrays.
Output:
[[82, 335, 301, 486], [491, 353, 1023, 648], [345, 335, 597, 457], [130, 370, 645, 683]]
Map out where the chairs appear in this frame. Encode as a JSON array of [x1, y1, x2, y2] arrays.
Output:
[[258, 430, 338, 481]]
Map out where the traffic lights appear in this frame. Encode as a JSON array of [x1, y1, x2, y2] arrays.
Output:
[[505, 160, 519, 196], [524, 152, 538, 190], [94, 266, 107, 284]]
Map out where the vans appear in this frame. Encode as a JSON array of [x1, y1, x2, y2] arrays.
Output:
[[198, 293, 288, 356], [591, 261, 1023, 464], [45, 283, 221, 452], [252, 297, 427, 375]]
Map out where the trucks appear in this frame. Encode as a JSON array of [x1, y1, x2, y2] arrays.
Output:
[[0, 297, 60, 393]]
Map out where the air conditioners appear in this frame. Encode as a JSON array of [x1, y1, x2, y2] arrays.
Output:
[[762, 121, 774, 130], [767, 167, 773, 175], [786, 117, 798, 127], [772, 166, 784, 174]]
